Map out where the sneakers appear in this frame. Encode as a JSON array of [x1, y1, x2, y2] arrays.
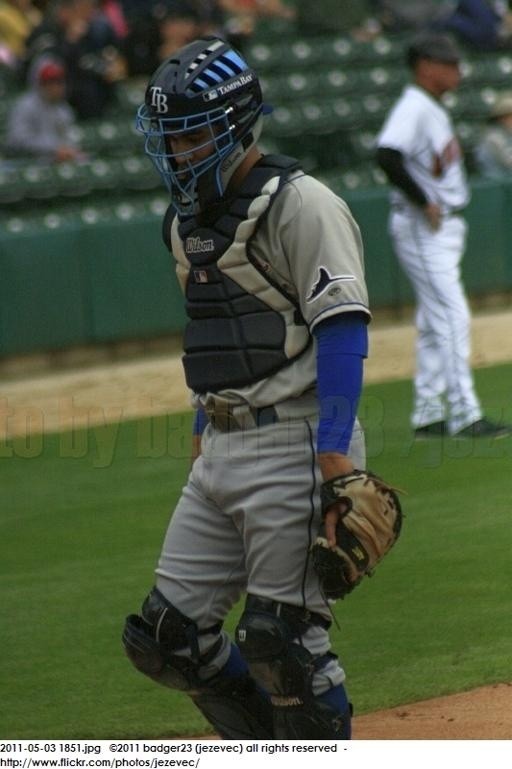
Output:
[[414, 421, 448, 439], [451, 418, 511, 442]]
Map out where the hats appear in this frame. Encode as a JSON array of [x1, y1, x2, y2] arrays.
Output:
[[405, 28, 461, 65]]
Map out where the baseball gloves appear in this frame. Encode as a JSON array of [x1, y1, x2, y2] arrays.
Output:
[[309, 469, 403, 598]]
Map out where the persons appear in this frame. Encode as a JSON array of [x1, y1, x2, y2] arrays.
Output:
[[375, 35, 512, 439], [121, 37, 400, 740]]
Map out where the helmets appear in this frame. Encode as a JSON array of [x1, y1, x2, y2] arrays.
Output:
[[134, 35, 272, 216]]
